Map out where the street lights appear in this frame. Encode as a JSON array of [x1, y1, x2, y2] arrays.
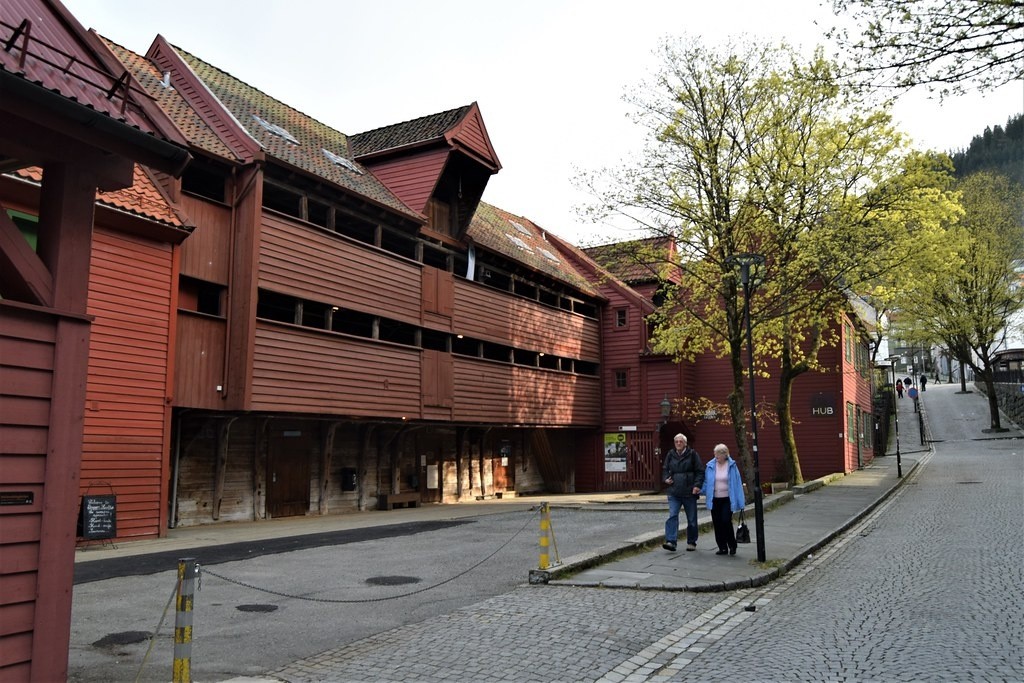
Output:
[[905, 350, 920, 414], [884, 356, 904, 477], [725, 251, 775, 564]]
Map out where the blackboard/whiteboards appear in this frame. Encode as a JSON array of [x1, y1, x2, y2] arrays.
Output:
[[82, 493, 117, 540]]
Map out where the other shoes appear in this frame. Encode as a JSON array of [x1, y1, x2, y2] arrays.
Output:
[[715, 547, 737, 555]]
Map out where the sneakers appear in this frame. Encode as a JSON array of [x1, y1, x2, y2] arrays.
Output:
[[662, 542, 677, 551], [686, 543, 697, 550]]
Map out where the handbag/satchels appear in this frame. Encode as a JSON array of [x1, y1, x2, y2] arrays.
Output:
[[737, 508, 750, 544]]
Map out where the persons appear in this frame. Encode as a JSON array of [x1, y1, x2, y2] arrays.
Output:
[[920, 373, 927, 391], [903, 377, 912, 392], [694, 443, 745, 556], [896, 378, 902, 386], [934, 370, 941, 384], [896, 382, 906, 398], [662, 433, 705, 552]]
